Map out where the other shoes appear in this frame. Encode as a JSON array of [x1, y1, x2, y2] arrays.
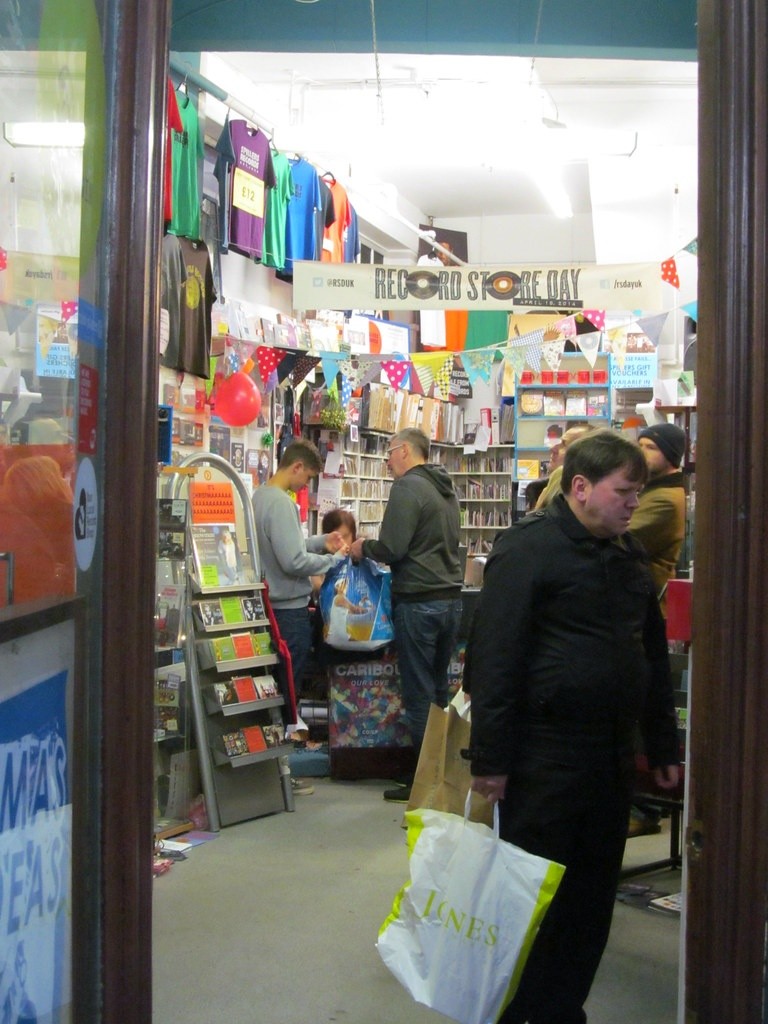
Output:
[[290, 778, 314, 795], [384, 787, 412, 803]]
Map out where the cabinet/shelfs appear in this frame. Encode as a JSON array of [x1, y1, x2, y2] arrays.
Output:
[[513, 351, 612, 480], [343, 430, 515, 557], [167, 451, 296, 833]]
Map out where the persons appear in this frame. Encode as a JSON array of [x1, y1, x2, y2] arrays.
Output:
[[623, 422, 690, 631], [1, 415, 82, 604], [244, 600, 257, 621], [325, 578, 371, 642], [351, 427, 464, 802], [458, 427, 683, 1022], [536, 424, 607, 474], [436, 239, 452, 268], [251, 439, 349, 795], [219, 528, 241, 583], [310, 510, 358, 593], [203, 603, 217, 626]]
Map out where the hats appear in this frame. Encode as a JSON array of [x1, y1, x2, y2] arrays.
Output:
[[638, 423, 686, 468]]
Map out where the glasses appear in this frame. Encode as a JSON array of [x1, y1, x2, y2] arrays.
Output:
[[387, 445, 403, 458]]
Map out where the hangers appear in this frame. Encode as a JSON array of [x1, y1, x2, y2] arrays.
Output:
[[247, 109, 258, 131], [174, 60, 193, 101], [288, 153, 300, 162], [428, 240, 436, 258], [322, 170, 336, 185]]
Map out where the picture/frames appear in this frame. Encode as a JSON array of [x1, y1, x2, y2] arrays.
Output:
[[201, 193, 222, 296]]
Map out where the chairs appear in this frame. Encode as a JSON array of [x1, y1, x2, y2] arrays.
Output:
[[616, 577, 692, 914]]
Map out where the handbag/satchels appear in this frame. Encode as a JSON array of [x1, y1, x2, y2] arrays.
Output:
[[400, 700, 494, 830], [319, 556, 396, 651], [374, 786, 566, 1024]]
